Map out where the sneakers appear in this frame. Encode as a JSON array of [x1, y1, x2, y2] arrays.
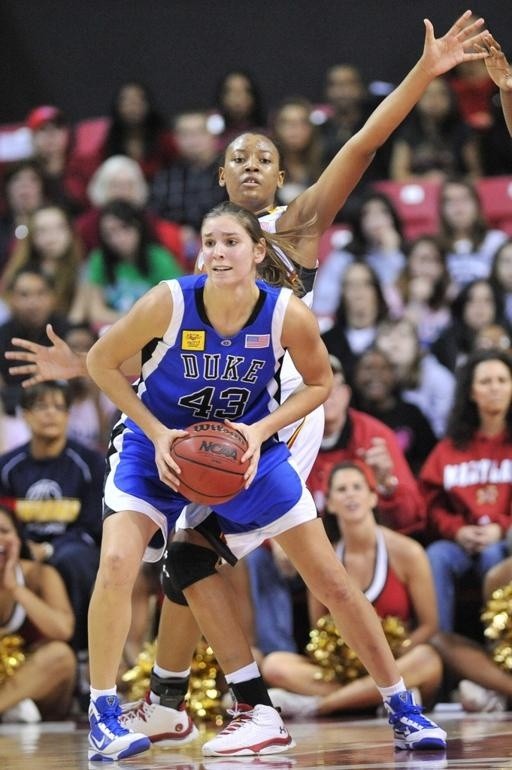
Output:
[[119, 695, 198, 750], [124, 754, 294, 770], [1, 696, 42, 725], [455, 678, 510, 714], [198, 700, 297, 762], [380, 684, 450, 751], [84, 693, 154, 764], [391, 750, 449, 770], [267, 687, 320, 721]]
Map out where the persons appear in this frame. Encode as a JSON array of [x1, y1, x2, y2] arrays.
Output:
[[0, 9, 512, 761]]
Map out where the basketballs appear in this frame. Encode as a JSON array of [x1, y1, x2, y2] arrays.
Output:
[[165, 421, 252, 506]]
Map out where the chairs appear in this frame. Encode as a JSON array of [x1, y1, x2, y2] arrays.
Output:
[[371, 179, 441, 243], [474, 175, 512, 236], [318, 223, 352, 264]]
